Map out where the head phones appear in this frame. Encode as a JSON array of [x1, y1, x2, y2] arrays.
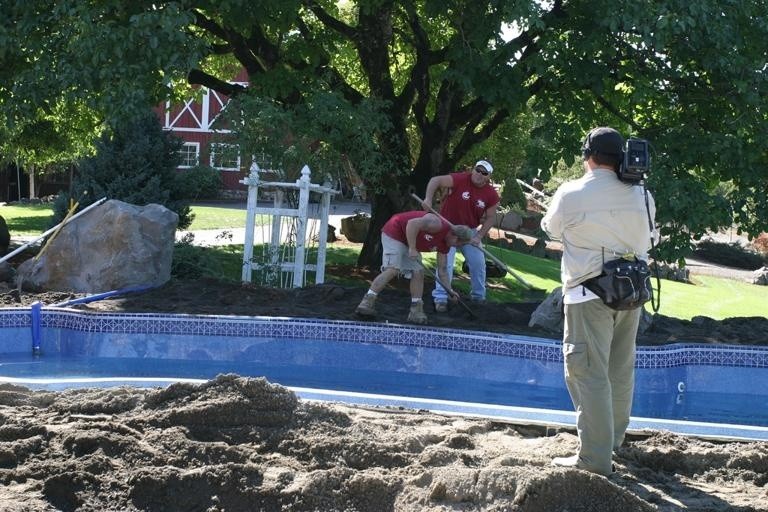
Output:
[[582, 127, 600, 161]]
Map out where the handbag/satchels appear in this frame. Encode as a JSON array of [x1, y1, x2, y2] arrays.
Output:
[[583, 257, 653, 310]]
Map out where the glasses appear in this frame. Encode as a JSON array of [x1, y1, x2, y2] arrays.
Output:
[[475, 167, 489, 176]]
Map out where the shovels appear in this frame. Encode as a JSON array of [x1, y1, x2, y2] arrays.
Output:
[[18, 190, 87, 293]]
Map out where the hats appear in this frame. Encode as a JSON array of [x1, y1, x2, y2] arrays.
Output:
[[476, 160, 493, 174]]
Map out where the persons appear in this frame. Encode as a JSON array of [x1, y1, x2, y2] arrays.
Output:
[[421, 160, 502, 314], [351, 211, 474, 325], [541, 126, 661, 475]]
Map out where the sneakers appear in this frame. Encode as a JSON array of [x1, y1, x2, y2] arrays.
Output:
[[354, 293, 376, 316], [551, 454, 579, 469], [406, 299, 428, 325], [435, 301, 447, 313]]
